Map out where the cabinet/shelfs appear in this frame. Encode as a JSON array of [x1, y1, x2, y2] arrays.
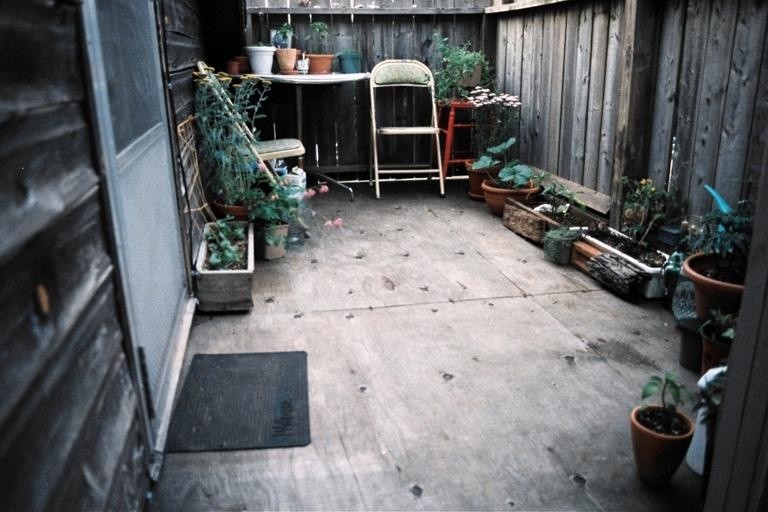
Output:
[[428, 97, 499, 197]]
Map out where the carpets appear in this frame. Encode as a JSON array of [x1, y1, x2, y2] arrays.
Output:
[[165, 351, 310, 454]]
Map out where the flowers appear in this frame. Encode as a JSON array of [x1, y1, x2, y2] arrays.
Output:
[[463, 85, 522, 165], [248, 157, 346, 248], [193, 63, 273, 206]]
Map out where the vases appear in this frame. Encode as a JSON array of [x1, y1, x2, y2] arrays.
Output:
[[258, 220, 290, 261], [214, 200, 248, 221], [569, 226, 672, 301], [465, 158, 501, 195]]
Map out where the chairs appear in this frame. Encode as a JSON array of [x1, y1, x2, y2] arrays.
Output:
[[197, 61, 312, 239], [369, 58, 446, 200]]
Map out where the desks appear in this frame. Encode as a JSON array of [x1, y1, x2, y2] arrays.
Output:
[[231, 71, 371, 202]]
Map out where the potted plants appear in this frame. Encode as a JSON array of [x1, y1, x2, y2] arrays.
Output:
[[464, 126, 612, 243], [429, 28, 499, 108], [246, 21, 363, 75], [195, 214, 257, 305]]
